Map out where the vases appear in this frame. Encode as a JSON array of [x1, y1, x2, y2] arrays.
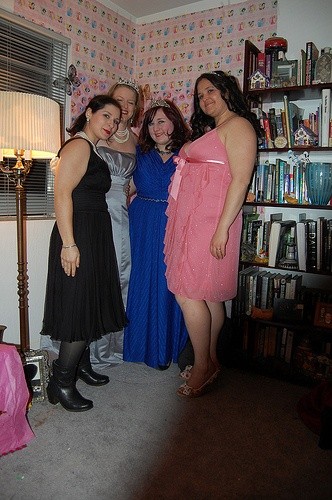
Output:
[[306, 162, 332, 206]]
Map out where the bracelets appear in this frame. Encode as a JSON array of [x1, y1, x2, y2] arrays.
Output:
[[62, 243, 76, 248]]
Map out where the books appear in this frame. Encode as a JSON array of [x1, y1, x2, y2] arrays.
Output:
[[247, 158, 308, 204], [247, 41, 319, 88], [241, 213, 332, 273], [242, 320, 295, 365], [251, 88, 332, 149], [236, 265, 332, 324]]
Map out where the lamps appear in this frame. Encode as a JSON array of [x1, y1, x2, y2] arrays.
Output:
[[0, 91, 61, 410]]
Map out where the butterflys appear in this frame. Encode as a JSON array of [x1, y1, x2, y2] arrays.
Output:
[[53, 65, 81, 95]]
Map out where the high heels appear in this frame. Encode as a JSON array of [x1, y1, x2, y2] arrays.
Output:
[[176, 361, 221, 400]]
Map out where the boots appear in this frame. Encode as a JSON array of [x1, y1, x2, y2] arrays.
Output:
[[45, 348, 110, 411]]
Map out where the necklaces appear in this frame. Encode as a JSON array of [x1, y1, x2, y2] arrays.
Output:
[[113, 127, 130, 144], [74, 131, 103, 159], [155, 145, 172, 156]]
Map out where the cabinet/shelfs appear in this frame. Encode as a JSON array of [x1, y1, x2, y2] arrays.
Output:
[[238, 39, 331, 390]]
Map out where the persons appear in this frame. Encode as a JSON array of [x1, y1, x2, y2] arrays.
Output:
[[163, 71, 266, 398], [123, 98, 191, 371], [40, 94, 130, 412], [49, 79, 141, 370]]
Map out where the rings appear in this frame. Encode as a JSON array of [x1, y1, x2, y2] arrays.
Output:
[[216, 249, 221, 250]]
